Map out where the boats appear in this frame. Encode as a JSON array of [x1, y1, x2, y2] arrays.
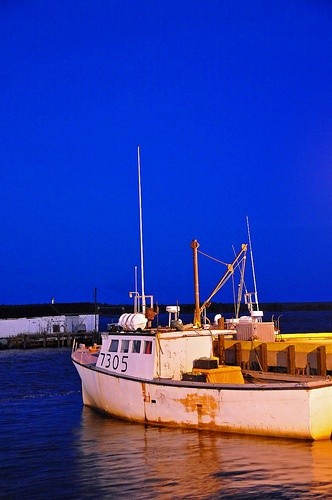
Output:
[[70, 144, 332, 440]]
